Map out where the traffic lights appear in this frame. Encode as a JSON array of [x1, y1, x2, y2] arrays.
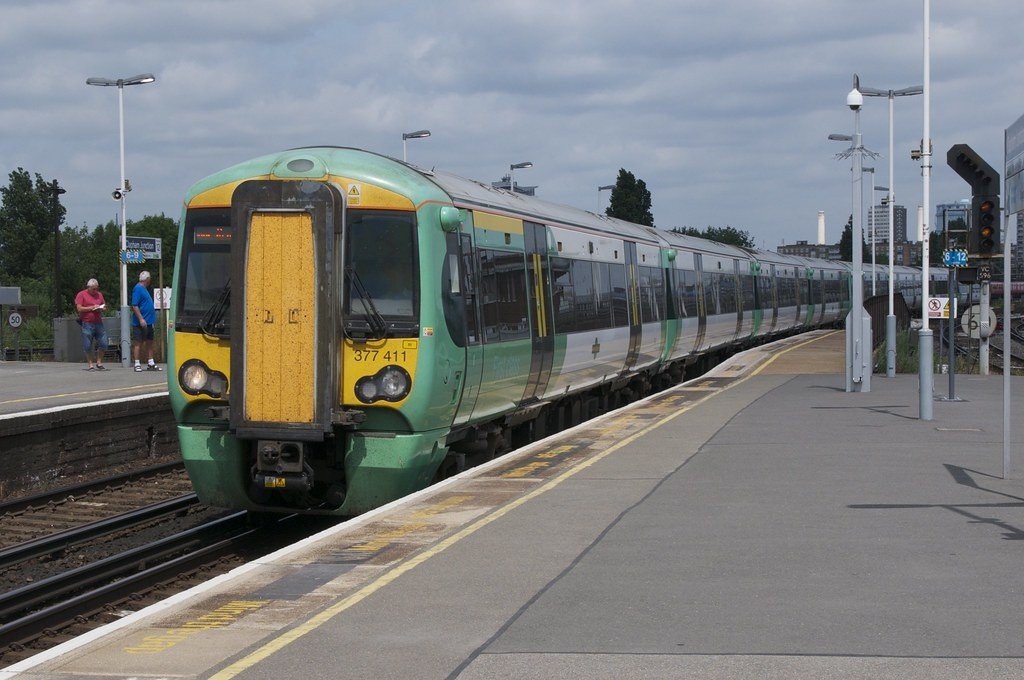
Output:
[[971, 196, 1001, 255], [944, 141, 1001, 196], [957, 266, 978, 284]]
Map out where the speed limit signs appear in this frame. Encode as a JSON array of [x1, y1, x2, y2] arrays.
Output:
[[8, 313, 22, 328]]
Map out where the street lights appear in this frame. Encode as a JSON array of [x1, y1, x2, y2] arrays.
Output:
[[509, 161, 534, 192], [850, 165, 876, 296], [49, 179, 66, 318], [402, 129, 432, 163], [597, 184, 617, 214], [857, 84, 923, 380], [85, 71, 156, 368]]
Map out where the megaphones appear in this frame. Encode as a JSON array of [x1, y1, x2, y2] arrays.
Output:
[[112, 190, 123, 200]]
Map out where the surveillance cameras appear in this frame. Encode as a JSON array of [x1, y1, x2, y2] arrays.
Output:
[[847, 88, 863, 110]]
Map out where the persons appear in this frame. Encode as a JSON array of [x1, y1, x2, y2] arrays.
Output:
[[75, 279, 109, 372], [131, 271, 162, 372]]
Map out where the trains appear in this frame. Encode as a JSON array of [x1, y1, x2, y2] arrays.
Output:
[[989, 279, 1024, 305], [162, 142, 979, 517]]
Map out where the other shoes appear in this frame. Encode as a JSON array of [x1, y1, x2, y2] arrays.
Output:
[[95, 363, 106, 370], [88, 367, 95, 372]]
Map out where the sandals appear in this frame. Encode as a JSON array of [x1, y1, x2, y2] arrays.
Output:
[[147, 364, 163, 371], [134, 366, 142, 372]]
